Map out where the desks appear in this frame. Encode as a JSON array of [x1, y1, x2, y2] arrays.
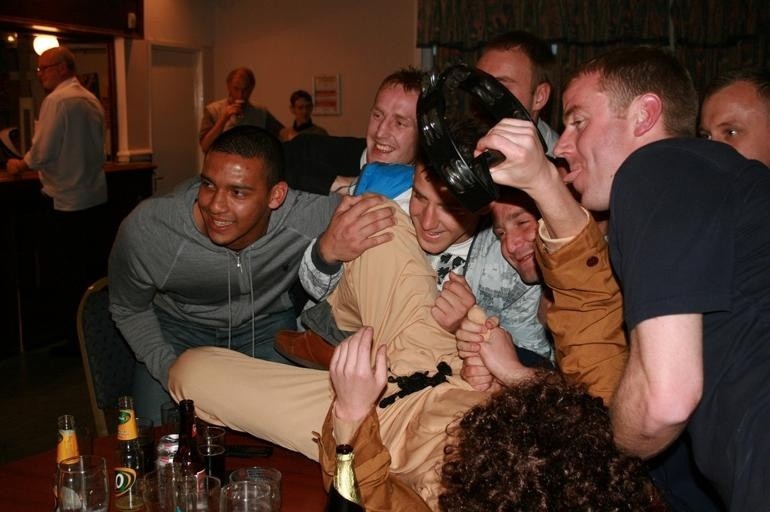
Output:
[[1, 419, 337, 511]]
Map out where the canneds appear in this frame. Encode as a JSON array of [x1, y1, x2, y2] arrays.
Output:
[[154, 433, 180, 510]]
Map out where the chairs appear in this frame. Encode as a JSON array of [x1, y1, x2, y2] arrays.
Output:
[[75, 276, 141, 440]]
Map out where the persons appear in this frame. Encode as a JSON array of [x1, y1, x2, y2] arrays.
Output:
[[106, 30, 770, 512], [6, 46, 107, 358]]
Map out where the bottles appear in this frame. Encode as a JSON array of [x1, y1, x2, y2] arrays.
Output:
[[112, 397, 145, 511], [53, 415, 85, 507], [323, 442, 367, 512]]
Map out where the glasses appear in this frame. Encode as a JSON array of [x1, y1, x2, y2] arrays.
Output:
[[34, 60, 63, 74]]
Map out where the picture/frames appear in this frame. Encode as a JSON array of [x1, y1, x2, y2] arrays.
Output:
[[312, 71, 341, 115]]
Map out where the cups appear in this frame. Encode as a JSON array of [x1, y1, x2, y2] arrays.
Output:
[[57, 453, 109, 511], [131, 416, 155, 460], [234, 100, 245, 119], [146, 399, 283, 512], [72, 421, 93, 455]]
